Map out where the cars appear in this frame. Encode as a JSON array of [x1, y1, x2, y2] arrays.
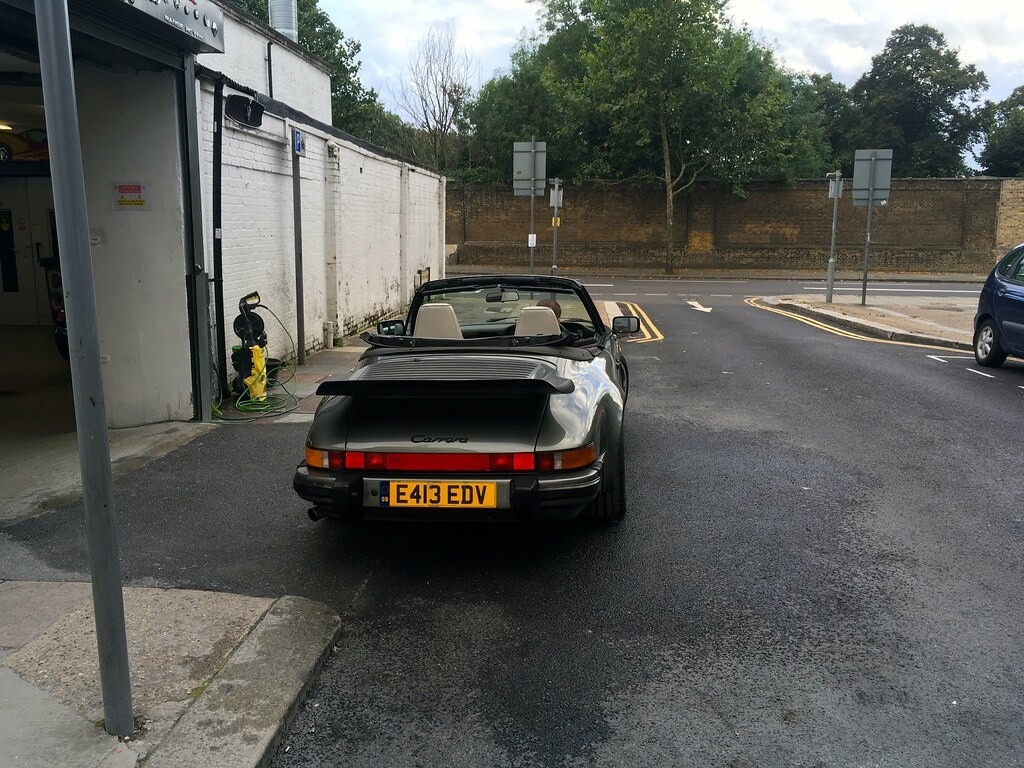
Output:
[[973, 243, 1024, 368]]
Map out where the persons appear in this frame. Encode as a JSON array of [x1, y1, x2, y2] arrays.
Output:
[[537, 298, 562, 327]]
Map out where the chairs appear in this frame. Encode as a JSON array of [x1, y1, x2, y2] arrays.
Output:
[[514, 305, 561, 337], [413, 303, 464, 340]]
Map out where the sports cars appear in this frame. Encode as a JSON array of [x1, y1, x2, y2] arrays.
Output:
[[293, 272, 640, 531]]
